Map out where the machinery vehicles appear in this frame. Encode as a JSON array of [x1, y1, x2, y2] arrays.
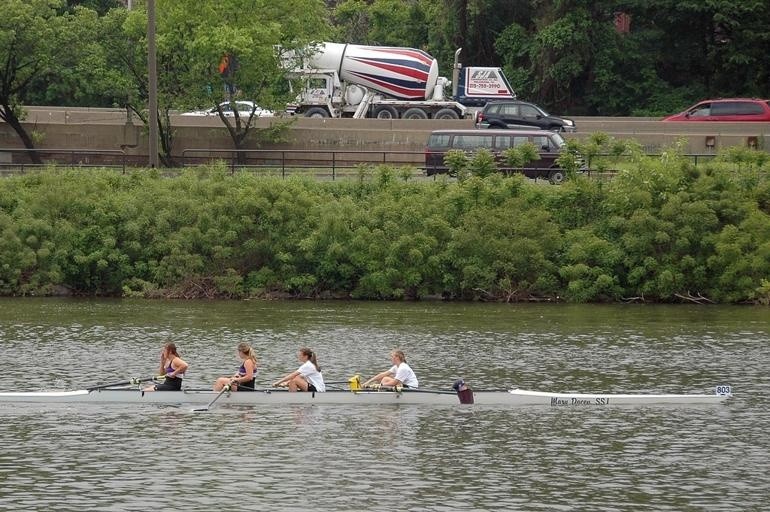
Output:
[[272, 40, 517, 119]]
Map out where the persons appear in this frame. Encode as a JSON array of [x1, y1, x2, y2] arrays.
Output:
[[139, 341, 188, 391], [269, 346, 327, 393], [212, 341, 259, 391], [362, 350, 419, 389]]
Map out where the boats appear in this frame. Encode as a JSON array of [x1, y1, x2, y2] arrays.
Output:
[[0, 386, 732, 411]]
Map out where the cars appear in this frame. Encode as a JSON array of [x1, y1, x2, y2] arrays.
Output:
[[473, 100, 576, 133], [663, 98, 770, 121], [181, 101, 280, 118]]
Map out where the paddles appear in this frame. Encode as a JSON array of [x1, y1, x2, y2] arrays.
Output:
[[193, 376, 239, 412], [324, 376, 360, 390], [86, 375, 172, 391], [367, 386, 474, 404]]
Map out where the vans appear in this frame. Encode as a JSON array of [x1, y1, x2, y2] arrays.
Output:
[[425, 128, 587, 184]]
